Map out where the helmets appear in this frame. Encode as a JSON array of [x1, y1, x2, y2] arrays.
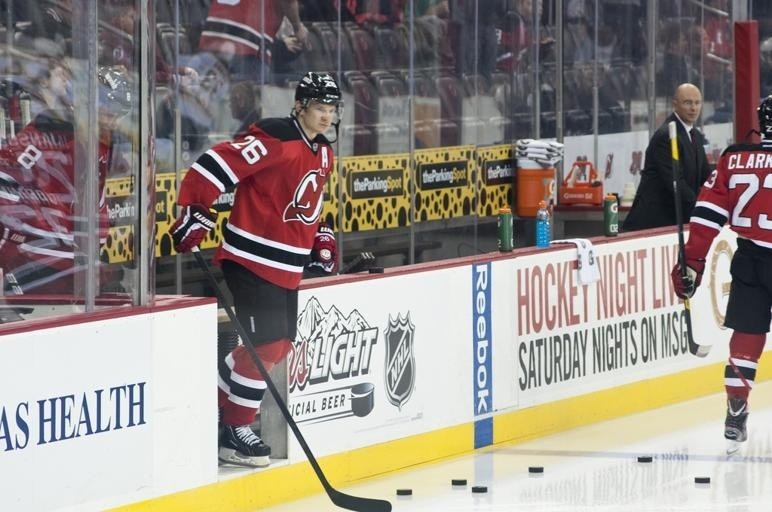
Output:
[[295, 71, 343, 109], [97, 66, 131, 117], [757, 95, 770, 139]]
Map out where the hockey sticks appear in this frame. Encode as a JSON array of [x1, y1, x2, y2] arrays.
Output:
[[191, 247, 391, 512]]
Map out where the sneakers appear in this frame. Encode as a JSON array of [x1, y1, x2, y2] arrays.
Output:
[[222, 424, 270, 458]]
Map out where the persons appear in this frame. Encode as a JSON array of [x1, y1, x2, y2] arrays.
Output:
[[671, 95, 772, 442], [0, 1, 771, 301], [168, 72, 343, 458]]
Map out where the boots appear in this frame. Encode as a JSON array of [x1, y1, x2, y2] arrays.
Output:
[[725, 396, 747, 442]]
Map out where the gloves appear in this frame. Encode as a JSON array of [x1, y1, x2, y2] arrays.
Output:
[[169, 203, 218, 254], [672, 247, 704, 298], [304, 223, 338, 277]]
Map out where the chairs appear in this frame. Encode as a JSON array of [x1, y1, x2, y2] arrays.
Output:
[[134, 18, 653, 174]]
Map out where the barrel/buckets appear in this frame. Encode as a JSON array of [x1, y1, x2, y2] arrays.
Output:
[[515, 159, 557, 217], [350, 383, 375, 417]]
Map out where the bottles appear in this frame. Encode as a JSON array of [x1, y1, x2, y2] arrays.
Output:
[[498, 205, 515, 251], [535, 201, 550, 248], [603, 193, 619, 236]]
